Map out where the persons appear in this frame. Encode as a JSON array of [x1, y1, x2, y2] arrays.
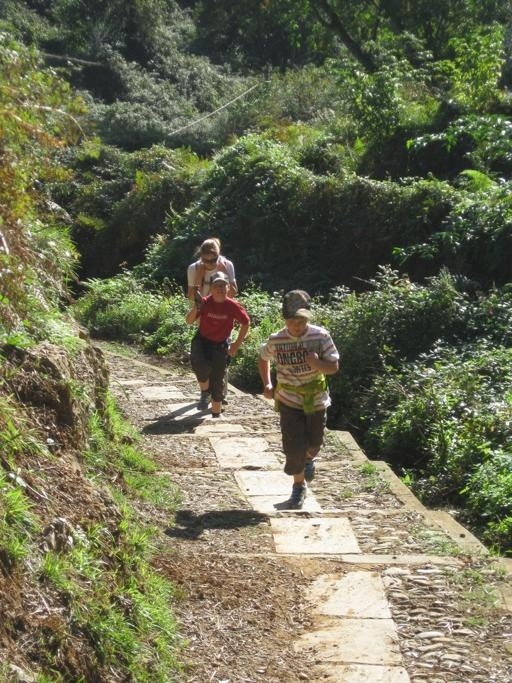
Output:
[[259, 289, 341, 509], [186, 237, 238, 413], [186, 272, 250, 419]]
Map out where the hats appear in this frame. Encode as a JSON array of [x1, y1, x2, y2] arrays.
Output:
[[283, 290, 312, 319], [210, 271, 230, 285]]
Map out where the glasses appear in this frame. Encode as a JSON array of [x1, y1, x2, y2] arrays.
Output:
[[200, 257, 218, 264]]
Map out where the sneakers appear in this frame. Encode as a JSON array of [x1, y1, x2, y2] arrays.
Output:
[[304, 457, 315, 482], [211, 401, 222, 415], [290, 482, 307, 508], [198, 392, 212, 410]]
